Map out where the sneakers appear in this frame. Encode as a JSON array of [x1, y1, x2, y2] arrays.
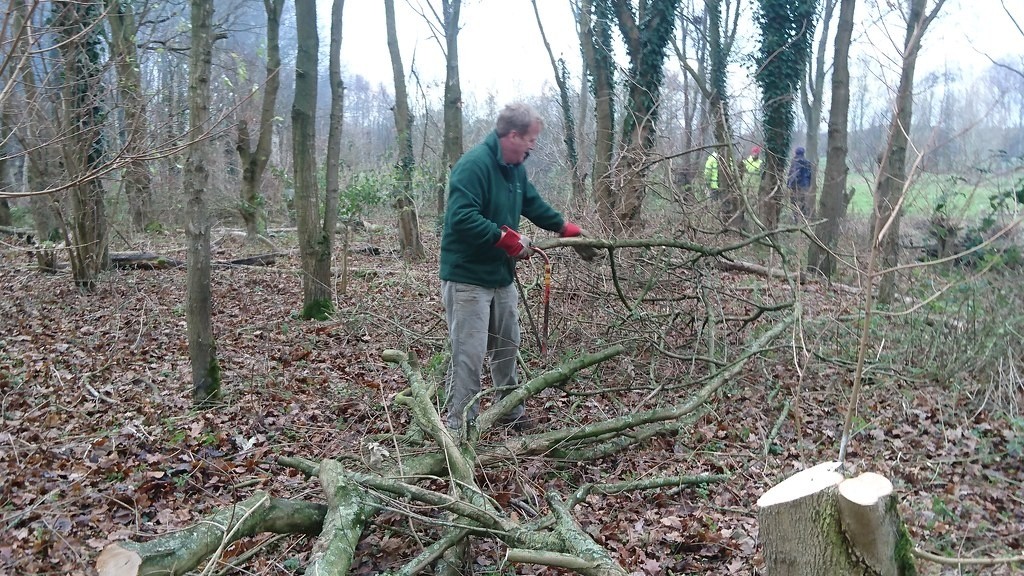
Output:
[[502, 416, 536, 434]]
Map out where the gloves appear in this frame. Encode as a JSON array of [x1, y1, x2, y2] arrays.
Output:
[[559, 224, 600, 263], [497, 227, 536, 262]]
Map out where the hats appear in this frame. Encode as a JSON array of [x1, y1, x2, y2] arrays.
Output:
[[751, 147, 761, 153], [795, 146, 805, 155]]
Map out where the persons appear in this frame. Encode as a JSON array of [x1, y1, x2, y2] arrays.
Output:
[[439, 103, 600, 437], [787, 147, 811, 224], [671, 157, 692, 203], [739, 149, 763, 196], [703, 147, 718, 202]]
